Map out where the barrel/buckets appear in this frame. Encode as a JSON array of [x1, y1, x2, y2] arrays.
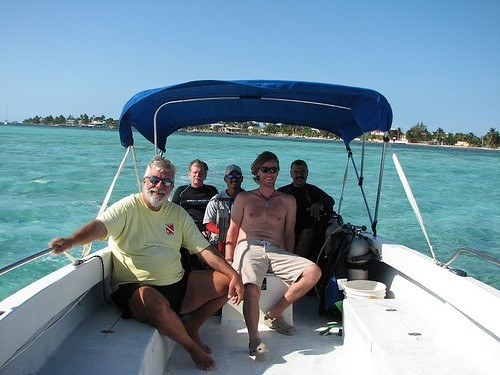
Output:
[[343, 280, 386, 300]]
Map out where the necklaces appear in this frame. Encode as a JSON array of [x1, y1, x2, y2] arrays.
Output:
[[258, 188, 275, 209]]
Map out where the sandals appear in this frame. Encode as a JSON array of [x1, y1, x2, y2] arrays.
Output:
[[264, 310, 295, 335], [249, 338, 267, 356]]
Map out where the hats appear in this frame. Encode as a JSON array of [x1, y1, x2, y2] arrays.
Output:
[[226, 165, 242, 175]]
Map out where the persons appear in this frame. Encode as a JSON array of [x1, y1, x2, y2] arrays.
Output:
[[49, 156, 245, 370], [225, 151, 321, 356], [203, 164, 248, 315], [277, 160, 335, 258], [172, 159, 219, 271]]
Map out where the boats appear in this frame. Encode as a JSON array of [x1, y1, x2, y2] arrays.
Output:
[[1, 74, 500, 375]]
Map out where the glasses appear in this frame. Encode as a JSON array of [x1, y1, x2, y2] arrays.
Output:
[[146, 176, 173, 187], [225, 175, 242, 180], [259, 167, 278, 173]]
